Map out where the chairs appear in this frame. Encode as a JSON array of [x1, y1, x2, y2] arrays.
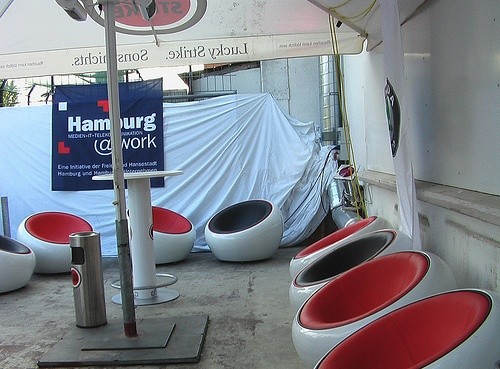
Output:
[[289, 215, 500, 369], [205, 199, 284, 261], [0, 235, 36, 293], [126, 205, 196, 265], [16, 211, 94, 274]]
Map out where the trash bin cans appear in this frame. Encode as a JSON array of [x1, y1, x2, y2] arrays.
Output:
[[69, 231, 107, 329]]
